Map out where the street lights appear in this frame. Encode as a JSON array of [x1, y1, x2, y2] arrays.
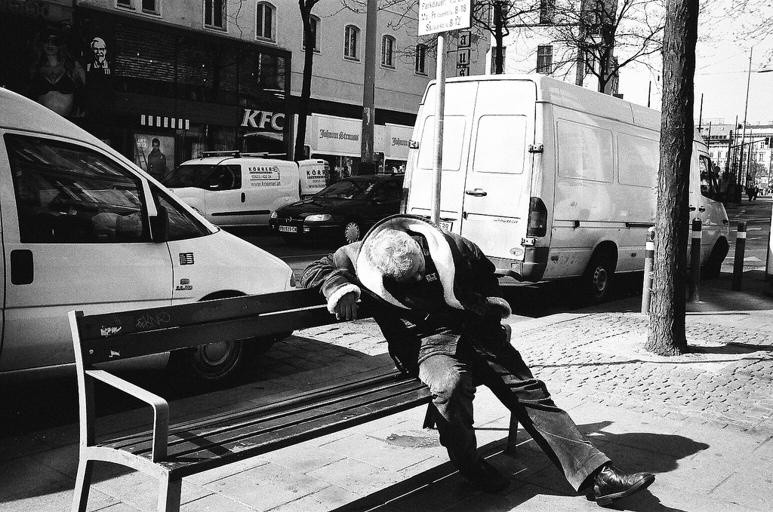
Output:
[[737, 46, 773, 185]]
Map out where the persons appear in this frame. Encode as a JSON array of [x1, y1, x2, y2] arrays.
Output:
[[86, 36, 109, 72], [29, 28, 86, 119], [301, 213, 654, 506], [146, 139, 167, 182]]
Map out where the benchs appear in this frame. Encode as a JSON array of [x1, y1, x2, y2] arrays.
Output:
[[66, 276, 520, 512]]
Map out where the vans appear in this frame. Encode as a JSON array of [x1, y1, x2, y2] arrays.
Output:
[[157, 149, 326, 231], [0, 84, 297, 390]]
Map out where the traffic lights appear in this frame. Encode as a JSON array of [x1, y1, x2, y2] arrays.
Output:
[[764, 137, 768, 145], [770, 138, 772, 148]]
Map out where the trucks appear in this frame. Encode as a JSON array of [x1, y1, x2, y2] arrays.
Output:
[[398, 72, 731, 303]]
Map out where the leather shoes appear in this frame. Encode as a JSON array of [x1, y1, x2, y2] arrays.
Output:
[[462, 457, 511, 498], [589, 459, 655, 506]]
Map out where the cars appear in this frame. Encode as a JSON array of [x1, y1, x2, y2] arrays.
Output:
[[268, 175, 404, 246]]
